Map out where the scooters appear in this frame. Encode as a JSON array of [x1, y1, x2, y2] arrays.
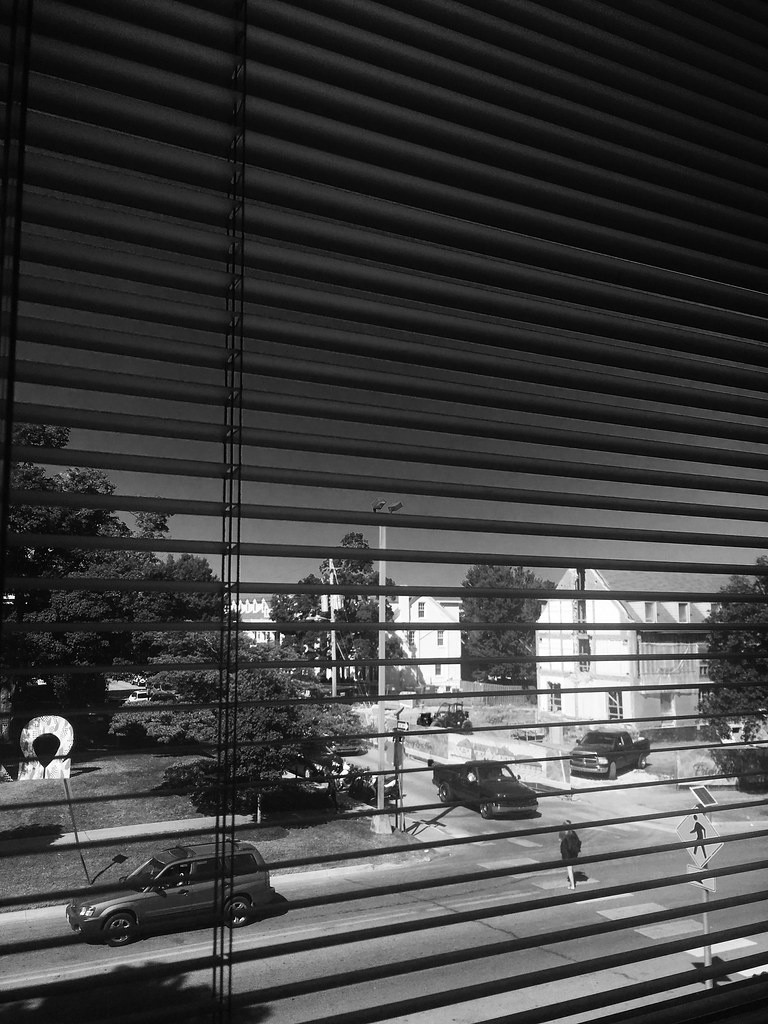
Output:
[[357, 767, 400, 801]]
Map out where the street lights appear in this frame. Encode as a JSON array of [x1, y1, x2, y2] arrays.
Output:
[[373, 497, 403, 832]]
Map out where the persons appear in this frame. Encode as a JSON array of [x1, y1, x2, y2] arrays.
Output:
[[557, 819, 577, 890], [163, 864, 186, 889]]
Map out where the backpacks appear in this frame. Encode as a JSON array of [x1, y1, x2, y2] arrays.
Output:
[[561, 836, 581, 856]]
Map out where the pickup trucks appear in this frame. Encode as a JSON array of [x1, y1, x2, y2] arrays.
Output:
[[434, 758, 539, 820], [567, 730, 651, 780]]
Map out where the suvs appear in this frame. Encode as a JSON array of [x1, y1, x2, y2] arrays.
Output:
[[67, 838, 276, 947], [416, 700, 472, 735], [128, 691, 150, 703]]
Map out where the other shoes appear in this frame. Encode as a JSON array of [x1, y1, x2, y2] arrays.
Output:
[[568, 887, 575, 890]]
